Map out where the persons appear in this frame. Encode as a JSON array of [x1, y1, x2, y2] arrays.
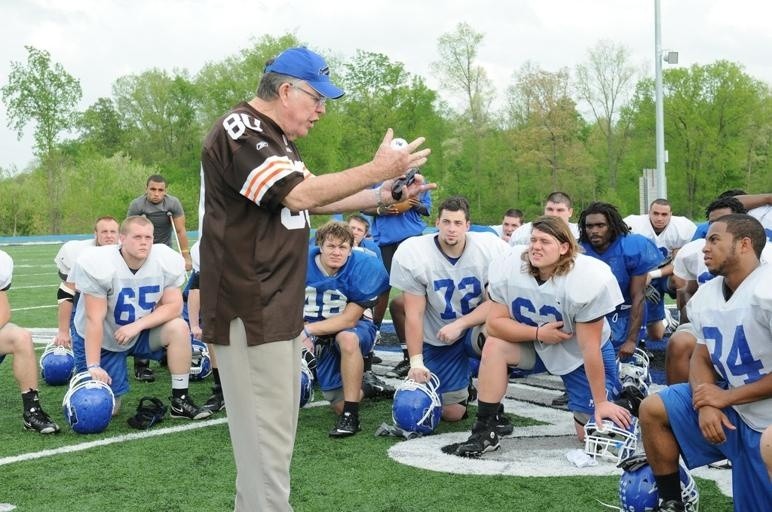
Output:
[[639, 214, 771, 511], [0, 251, 60, 434], [127, 174, 192, 381], [298, 191, 772, 438], [200, 45, 438, 510], [54, 214, 225, 418], [456, 216, 645, 454]]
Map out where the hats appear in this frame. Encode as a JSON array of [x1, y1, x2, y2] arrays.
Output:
[[264, 46, 345, 100]]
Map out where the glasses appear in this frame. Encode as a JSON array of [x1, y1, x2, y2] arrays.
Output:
[[616, 347, 652, 397], [300, 358, 314, 409], [62, 371, 116, 434], [392, 372, 442, 434], [583, 415, 641, 463], [189, 339, 212, 381], [618, 458, 700, 512], [290, 82, 325, 106], [40, 340, 74, 386]]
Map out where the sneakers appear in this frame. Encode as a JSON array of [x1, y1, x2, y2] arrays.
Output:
[[134, 359, 154, 381], [364, 369, 395, 396], [651, 496, 685, 512], [552, 392, 569, 404], [169, 386, 225, 420], [395, 352, 411, 368], [23, 406, 60, 434], [456, 403, 512, 457], [329, 410, 362, 436], [664, 308, 678, 331]]
